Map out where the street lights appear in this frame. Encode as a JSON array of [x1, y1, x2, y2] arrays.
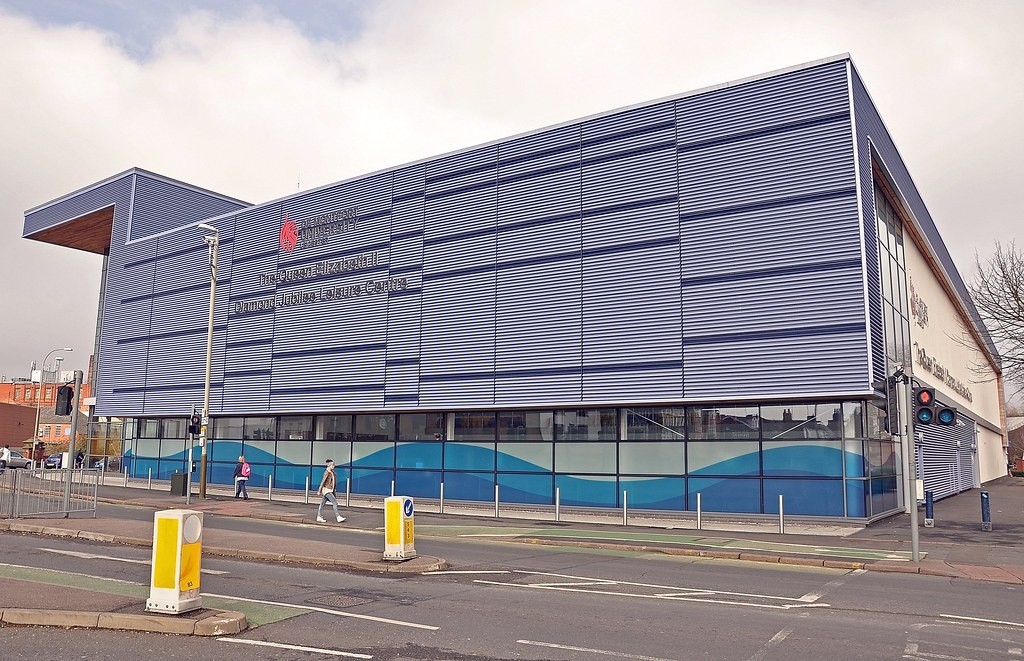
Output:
[[30, 347, 73, 469]]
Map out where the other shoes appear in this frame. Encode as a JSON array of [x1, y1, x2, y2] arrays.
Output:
[[244, 498, 248, 500], [235, 496, 238, 498]]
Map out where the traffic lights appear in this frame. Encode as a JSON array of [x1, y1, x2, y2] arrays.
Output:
[[188, 415, 200, 434], [870, 376, 898, 435], [913, 387, 958, 427]]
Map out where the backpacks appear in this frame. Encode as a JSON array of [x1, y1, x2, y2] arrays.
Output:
[[242, 462, 250, 477]]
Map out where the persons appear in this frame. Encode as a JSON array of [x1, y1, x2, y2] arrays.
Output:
[[316, 459, 347, 523], [75, 447, 84, 468], [0, 445, 11, 475], [232, 456, 249, 500]]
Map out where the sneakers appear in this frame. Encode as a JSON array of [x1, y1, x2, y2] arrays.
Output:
[[316, 515, 327, 523], [337, 515, 347, 522]]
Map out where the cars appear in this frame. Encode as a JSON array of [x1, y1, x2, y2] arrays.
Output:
[[45, 454, 63, 470], [5, 451, 31, 469], [95, 457, 120, 471]]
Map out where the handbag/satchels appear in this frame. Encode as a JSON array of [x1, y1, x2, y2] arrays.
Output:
[[0, 448, 4, 458]]
